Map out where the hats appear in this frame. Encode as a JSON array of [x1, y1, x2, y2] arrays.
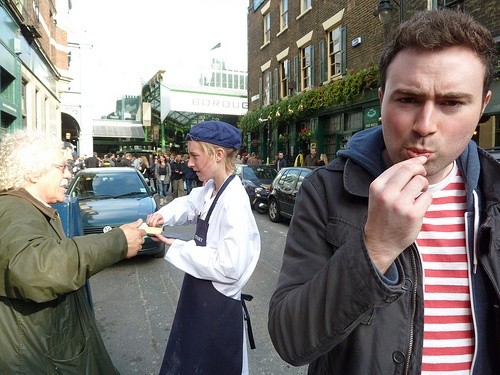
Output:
[[184, 120, 242, 150]]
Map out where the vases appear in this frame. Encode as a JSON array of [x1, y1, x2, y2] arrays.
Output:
[[302, 136, 307, 140]]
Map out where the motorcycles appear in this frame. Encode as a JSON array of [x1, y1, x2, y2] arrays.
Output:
[[252, 188, 269, 214]]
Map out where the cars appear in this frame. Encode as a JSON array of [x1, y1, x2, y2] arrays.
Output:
[[66, 167, 166, 258], [269, 167, 313, 224], [234, 164, 278, 214]]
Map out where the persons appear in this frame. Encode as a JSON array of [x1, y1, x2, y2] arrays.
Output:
[[146, 121, 261, 375], [268, 9, 500, 375], [236, 147, 329, 166], [1, 127, 206, 375]]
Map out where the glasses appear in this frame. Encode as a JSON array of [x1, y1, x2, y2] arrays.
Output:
[[55, 163, 67, 173]]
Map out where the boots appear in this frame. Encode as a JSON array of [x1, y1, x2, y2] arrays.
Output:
[[160, 197, 169, 206]]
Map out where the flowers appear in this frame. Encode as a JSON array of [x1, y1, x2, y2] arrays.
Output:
[[299, 128, 311, 137]]
